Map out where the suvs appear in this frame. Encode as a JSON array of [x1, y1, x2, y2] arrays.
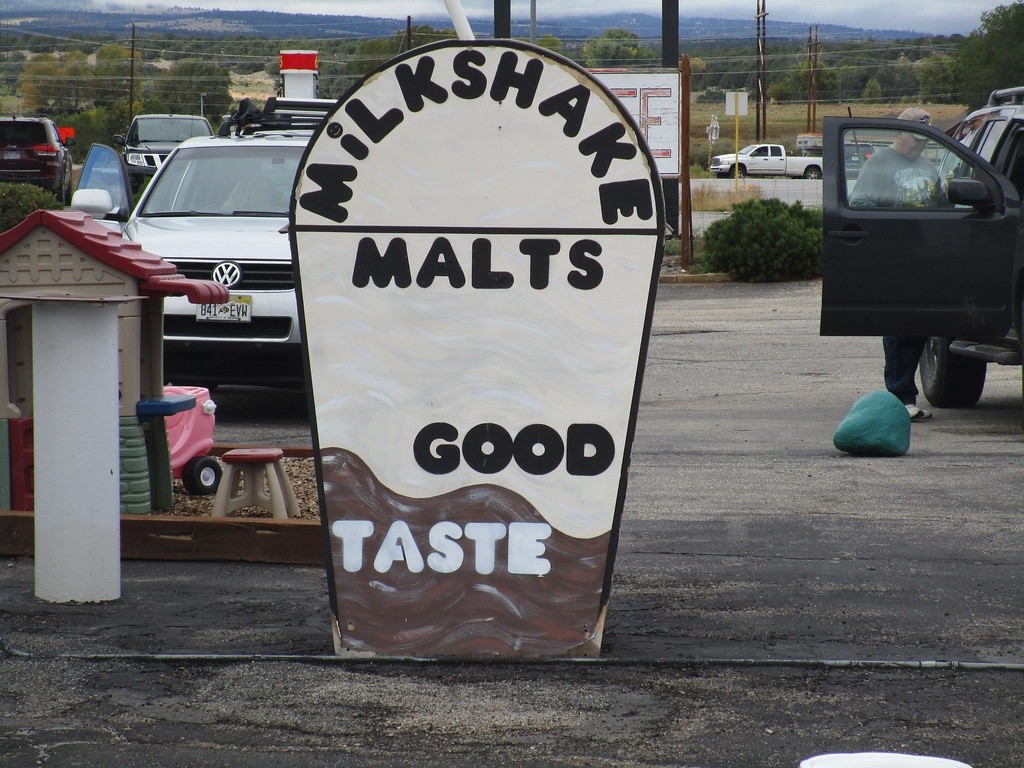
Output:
[[820, 87, 1024, 407], [113, 112, 218, 191], [0, 116, 74, 204]]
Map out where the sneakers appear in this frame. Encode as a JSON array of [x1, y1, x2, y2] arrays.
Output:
[[906, 404, 932, 420]]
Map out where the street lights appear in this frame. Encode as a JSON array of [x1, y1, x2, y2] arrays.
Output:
[[200, 92, 206, 118]]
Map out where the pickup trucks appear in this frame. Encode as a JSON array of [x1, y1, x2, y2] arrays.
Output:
[[711, 142, 824, 179]]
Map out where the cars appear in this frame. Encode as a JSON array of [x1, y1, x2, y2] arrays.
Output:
[[843, 143, 875, 179], [72, 131, 317, 400]]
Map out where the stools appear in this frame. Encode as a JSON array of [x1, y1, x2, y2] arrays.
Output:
[[211, 447, 302, 521]]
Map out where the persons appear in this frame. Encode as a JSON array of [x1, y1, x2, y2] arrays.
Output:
[[848, 108, 949, 419], [220, 163, 289, 209]]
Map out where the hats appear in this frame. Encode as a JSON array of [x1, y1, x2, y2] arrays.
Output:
[[897, 108, 934, 139]]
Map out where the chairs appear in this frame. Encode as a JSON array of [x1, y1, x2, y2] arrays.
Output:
[[141, 125, 157, 140], [220, 164, 290, 212]]
